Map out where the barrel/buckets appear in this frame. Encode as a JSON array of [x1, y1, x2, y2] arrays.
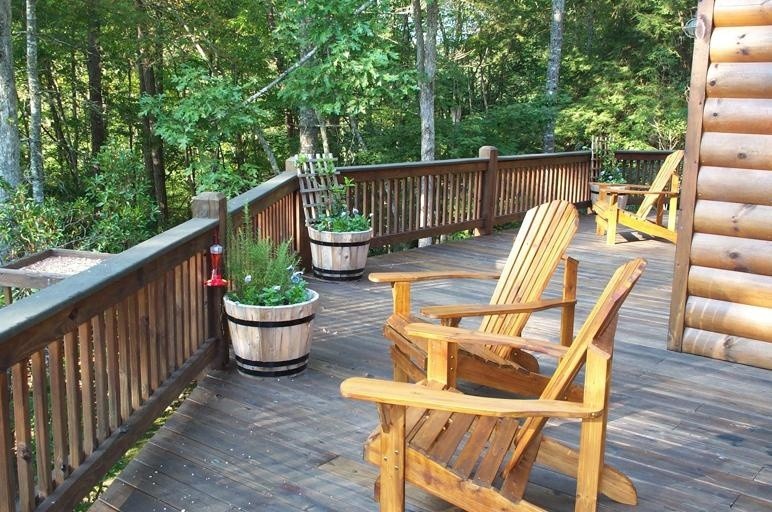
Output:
[[307, 224, 374, 280], [590, 184, 630, 214], [221, 288, 320, 379]]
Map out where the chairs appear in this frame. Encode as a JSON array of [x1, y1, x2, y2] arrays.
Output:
[[588, 149, 685, 246]]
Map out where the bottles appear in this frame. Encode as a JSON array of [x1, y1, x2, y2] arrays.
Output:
[[210, 230, 223, 282]]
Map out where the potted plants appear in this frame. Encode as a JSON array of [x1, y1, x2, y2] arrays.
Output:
[[308, 174, 375, 280], [223, 197, 320, 381]]
